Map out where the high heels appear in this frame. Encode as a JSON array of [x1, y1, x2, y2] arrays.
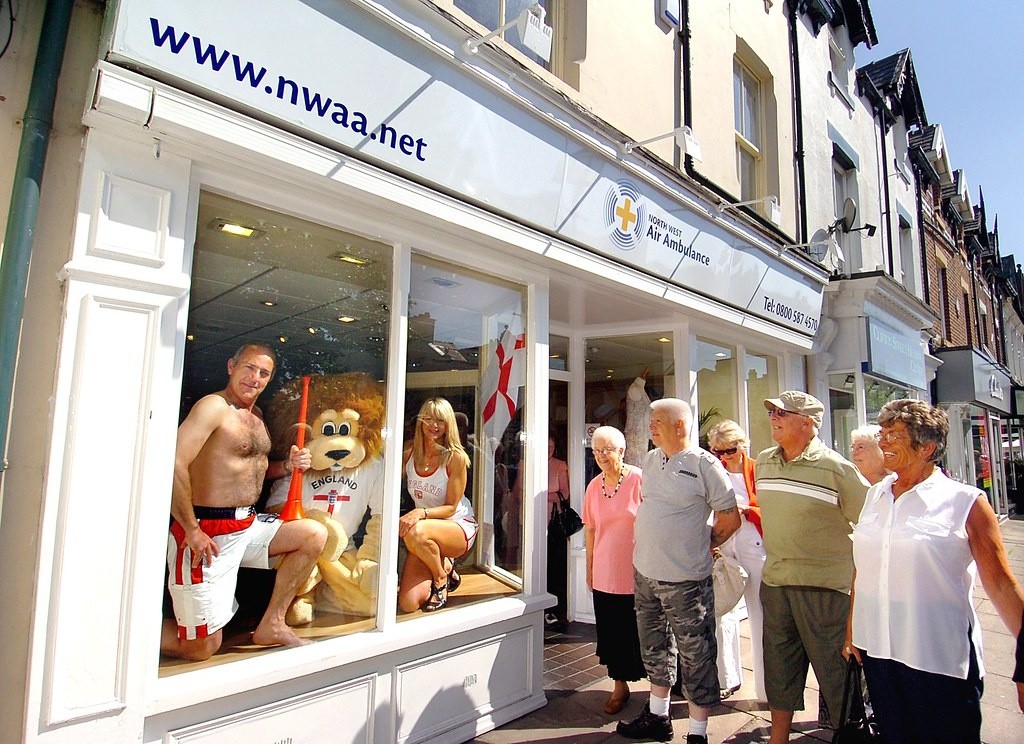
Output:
[[447, 557, 463, 592], [426, 577, 449, 611]]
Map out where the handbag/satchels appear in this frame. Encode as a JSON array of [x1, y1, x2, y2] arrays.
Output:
[[832, 655, 882, 744], [547, 492, 584, 542], [712, 548, 750, 618]]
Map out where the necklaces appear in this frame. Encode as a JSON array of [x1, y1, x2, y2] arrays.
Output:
[[602, 464, 624, 498], [423, 453, 436, 472]]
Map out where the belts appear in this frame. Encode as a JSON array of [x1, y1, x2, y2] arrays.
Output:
[[192, 505, 254, 520]]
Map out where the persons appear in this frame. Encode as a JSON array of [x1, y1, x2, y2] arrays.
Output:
[[408, 412, 527, 570], [754, 390, 872, 744], [157, 343, 328, 662], [842, 399, 1024, 744], [707, 420, 767, 702], [513, 434, 570, 623], [581, 426, 654, 713], [974, 451, 989, 491], [616, 398, 742, 744], [399, 398, 479, 613]]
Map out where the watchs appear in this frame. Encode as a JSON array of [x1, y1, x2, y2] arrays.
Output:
[[423, 507, 429, 520], [283, 460, 292, 476]]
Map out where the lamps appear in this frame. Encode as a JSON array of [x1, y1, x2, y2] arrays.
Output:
[[717, 194, 781, 226], [778, 238, 845, 263], [623, 126, 703, 163], [461, 3, 553, 63], [990, 362, 1011, 375], [842, 374, 909, 399]]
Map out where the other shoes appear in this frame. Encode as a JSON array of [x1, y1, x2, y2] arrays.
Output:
[[719, 684, 740, 698], [605, 690, 629, 713]]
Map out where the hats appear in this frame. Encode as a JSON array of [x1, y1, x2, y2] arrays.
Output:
[[764, 391, 824, 429]]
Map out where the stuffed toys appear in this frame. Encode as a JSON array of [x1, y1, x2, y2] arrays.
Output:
[[265, 372, 386, 626]]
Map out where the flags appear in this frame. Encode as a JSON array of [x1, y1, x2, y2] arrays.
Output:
[[478, 319, 526, 456]]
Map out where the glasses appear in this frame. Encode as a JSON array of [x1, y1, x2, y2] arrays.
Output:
[[848, 443, 877, 451], [874, 433, 914, 443], [592, 447, 620, 455], [768, 409, 808, 418], [714, 442, 739, 455]]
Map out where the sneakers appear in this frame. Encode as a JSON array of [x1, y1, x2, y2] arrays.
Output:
[[683, 732, 708, 744], [616, 703, 674, 742]]
